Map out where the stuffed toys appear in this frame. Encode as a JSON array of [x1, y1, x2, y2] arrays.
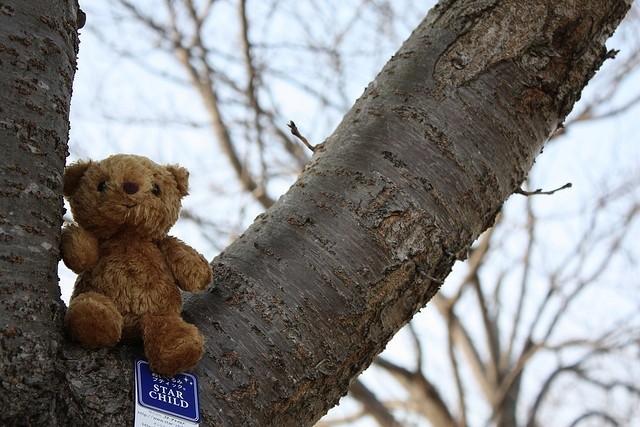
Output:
[[59, 153, 212, 376]]
